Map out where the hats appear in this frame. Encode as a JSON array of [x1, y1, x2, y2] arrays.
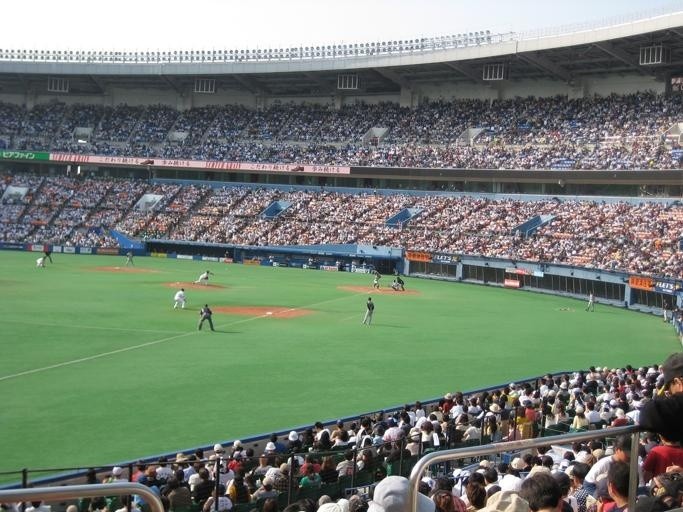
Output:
[[111, 365, 683, 512]]
